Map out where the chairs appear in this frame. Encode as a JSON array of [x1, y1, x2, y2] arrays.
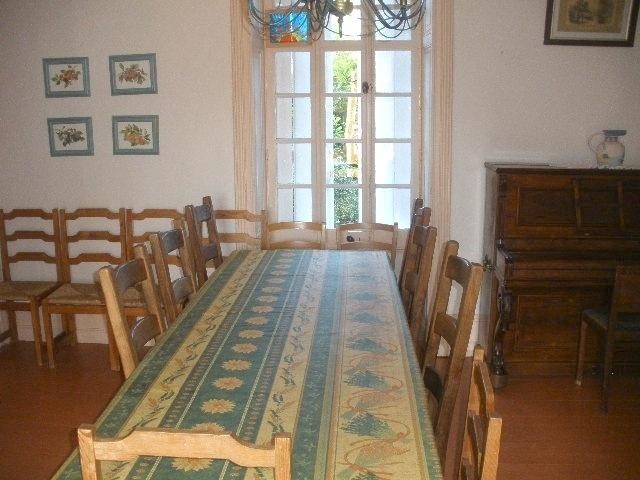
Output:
[[149, 216, 200, 328], [41, 205, 124, 375], [93, 241, 169, 379], [124, 207, 188, 321], [422, 240, 485, 477], [569, 264, 638, 414], [184, 194, 224, 289], [1, 206, 68, 366], [188, 201, 268, 282], [402, 215, 439, 348], [333, 219, 401, 271], [73, 417, 298, 479], [454, 341, 508, 476], [397, 195, 426, 299], [265, 218, 327, 251]]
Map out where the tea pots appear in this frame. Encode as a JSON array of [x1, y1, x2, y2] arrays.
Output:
[[586, 129, 627, 169]]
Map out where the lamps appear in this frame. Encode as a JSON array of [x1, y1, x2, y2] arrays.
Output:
[[241, 0, 426, 44]]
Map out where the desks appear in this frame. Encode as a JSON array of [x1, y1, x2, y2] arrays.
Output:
[[51, 248, 450, 478]]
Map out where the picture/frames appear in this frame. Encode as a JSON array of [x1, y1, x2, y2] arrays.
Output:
[[47, 117, 93, 158], [111, 115, 159, 156], [109, 53, 158, 96], [544, 0, 640, 48], [43, 58, 91, 97]]
